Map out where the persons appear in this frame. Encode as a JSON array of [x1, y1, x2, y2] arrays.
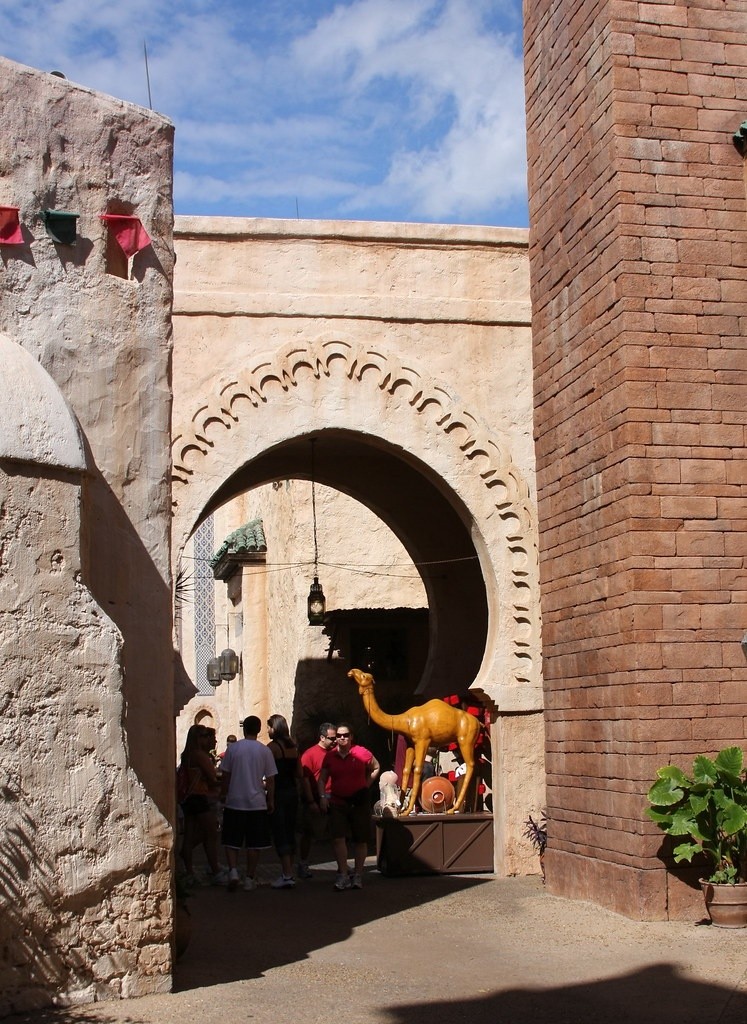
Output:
[[176, 724, 239, 887], [451, 756, 466, 779], [264, 713, 304, 889], [294, 722, 348, 883], [421, 745, 441, 783], [317, 722, 380, 888], [218, 715, 278, 891]]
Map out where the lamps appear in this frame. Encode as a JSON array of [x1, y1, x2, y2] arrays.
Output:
[[206, 658, 224, 689], [307, 482, 326, 625], [218, 649, 240, 684]]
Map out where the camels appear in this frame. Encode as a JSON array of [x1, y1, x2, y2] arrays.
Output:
[[348, 669, 486, 817]]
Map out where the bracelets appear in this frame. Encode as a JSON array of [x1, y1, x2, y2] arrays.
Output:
[[307, 799, 316, 805]]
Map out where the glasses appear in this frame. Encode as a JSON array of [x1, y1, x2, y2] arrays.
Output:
[[336, 733, 350, 738], [325, 737, 336, 741]]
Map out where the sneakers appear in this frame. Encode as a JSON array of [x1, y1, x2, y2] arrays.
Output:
[[350, 876, 363, 889], [333, 876, 351, 889], [299, 864, 312, 878], [243, 874, 261, 891], [210, 871, 228, 883], [228, 867, 242, 881], [271, 873, 297, 888]]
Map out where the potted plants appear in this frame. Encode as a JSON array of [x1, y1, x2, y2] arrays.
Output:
[[645, 746, 747, 930]]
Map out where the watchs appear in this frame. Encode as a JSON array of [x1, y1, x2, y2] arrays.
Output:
[[319, 794, 326, 798]]
